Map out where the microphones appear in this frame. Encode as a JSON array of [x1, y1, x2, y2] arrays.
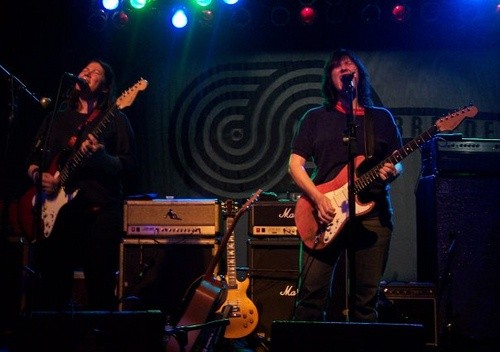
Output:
[[40, 97, 53, 106], [64, 73, 87, 90], [341, 71, 354, 82]]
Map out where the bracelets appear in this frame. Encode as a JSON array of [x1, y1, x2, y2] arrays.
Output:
[[31, 168, 38, 179]]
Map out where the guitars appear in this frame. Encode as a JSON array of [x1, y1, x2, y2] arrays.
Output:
[[294, 102, 478, 251], [220, 200, 258, 339], [8, 77, 148, 245], [165, 189, 262, 352]]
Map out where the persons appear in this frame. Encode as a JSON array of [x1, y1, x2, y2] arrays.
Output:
[[0, 58, 136, 308], [287, 48, 403, 323]]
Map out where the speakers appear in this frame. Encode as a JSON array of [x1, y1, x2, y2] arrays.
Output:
[[116, 237, 224, 327], [269, 320, 424, 352], [25, 308, 166, 352], [379, 285, 445, 349], [414, 173, 500, 351], [245, 238, 305, 346]]
